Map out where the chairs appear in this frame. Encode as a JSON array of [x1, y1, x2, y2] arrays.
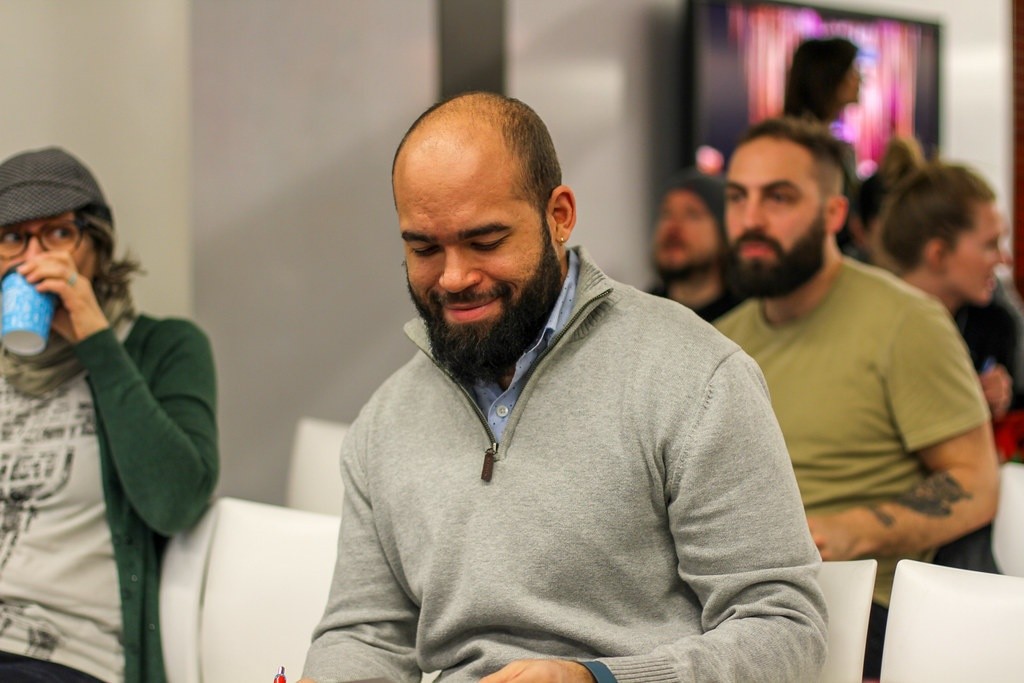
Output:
[[159, 418, 1024, 683]]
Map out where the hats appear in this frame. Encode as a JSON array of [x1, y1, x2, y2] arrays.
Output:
[[0, 147, 109, 227]]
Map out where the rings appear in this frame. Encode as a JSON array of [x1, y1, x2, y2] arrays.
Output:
[[67, 271, 78, 286]]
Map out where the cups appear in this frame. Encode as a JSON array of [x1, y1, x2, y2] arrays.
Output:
[[0, 264, 57, 356]]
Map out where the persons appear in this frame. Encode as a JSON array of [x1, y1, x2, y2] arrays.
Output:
[[643, 171, 746, 323], [296, 91, 830, 683], [0, 147, 218, 683], [711, 118, 1000, 683], [783, 37, 1024, 574]]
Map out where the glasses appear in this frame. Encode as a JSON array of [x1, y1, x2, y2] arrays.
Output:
[[2, 219, 86, 260]]
